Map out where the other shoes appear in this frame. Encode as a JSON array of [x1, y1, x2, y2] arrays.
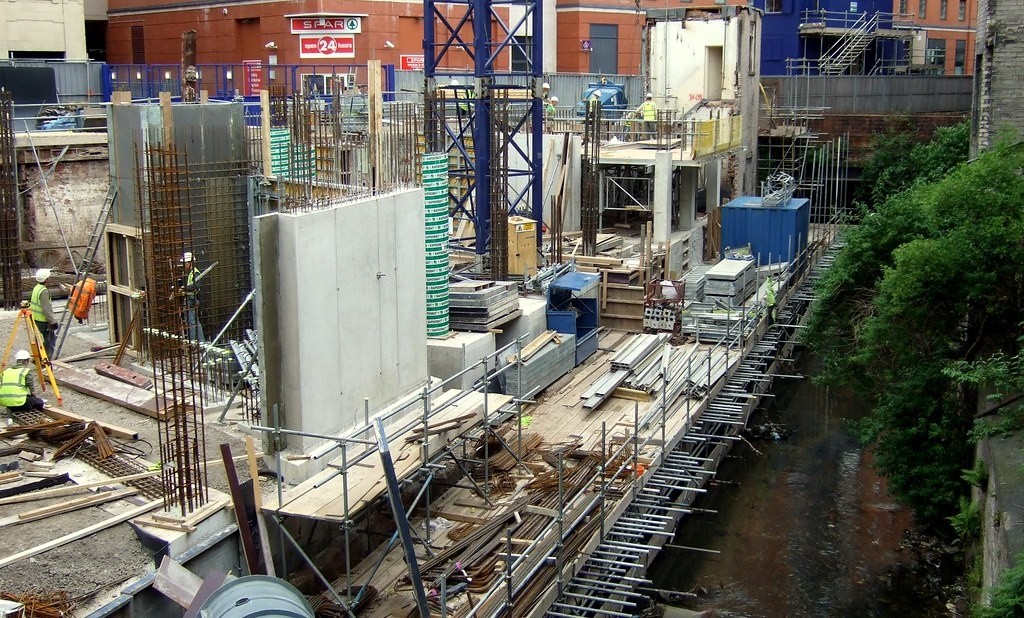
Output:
[[6, 408, 12, 417], [31, 357, 45, 369]]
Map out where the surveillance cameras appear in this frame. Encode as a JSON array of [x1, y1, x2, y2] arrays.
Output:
[[386, 41, 395, 48], [265, 42, 274, 48]]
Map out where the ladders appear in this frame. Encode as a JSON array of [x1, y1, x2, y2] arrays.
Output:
[[50, 184, 118, 361]]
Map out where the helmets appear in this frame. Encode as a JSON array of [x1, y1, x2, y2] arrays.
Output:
[[542, 83, 550, 89], [594, 90, 602, 97], [180, 252, 196, 262], [450, 80, 460, 88], [35, 268, 52, 282], [646, 93, 652, 98], [627, 112, 636, 118], [551, 96, 559, 102], [15, 350, 30, 359]]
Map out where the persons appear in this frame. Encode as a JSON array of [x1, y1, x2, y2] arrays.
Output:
[[542, 82, 551, 106], [619, 92, 657, 142], [586, 90, 602, 125], [461, 83, 475, 115], [546, 96, 558, 131], [29, 268, 58, 365], [180, 252, 206, 342], [0, 349, 44, 411]]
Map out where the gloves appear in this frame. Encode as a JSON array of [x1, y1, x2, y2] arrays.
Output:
[[52, 323, 58, 330]]
